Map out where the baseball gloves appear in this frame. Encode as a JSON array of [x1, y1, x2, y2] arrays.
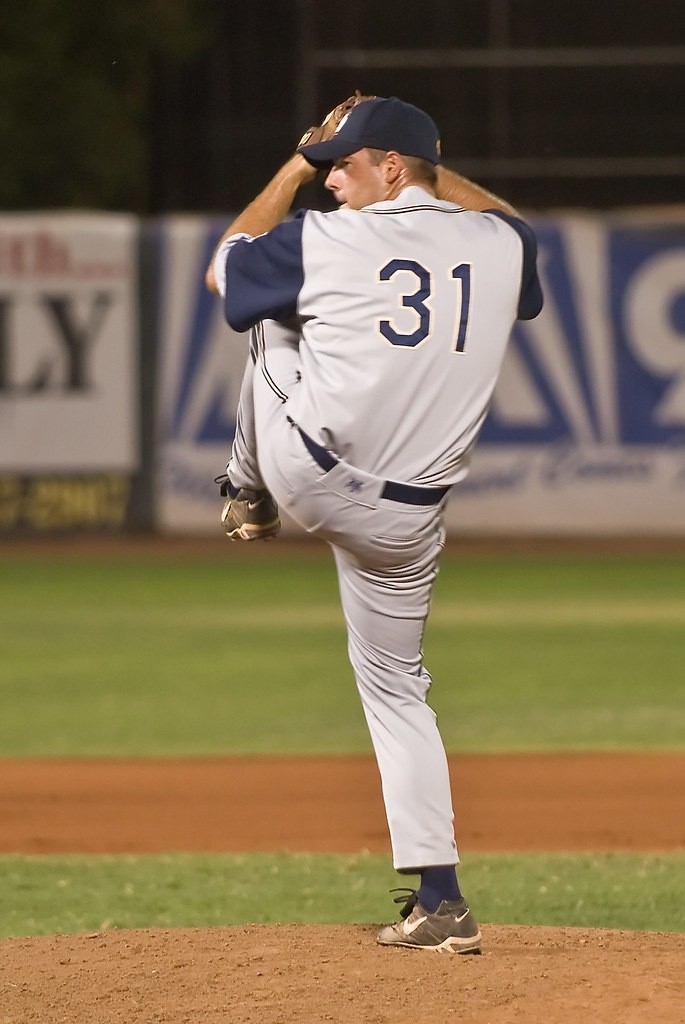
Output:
[[297, 96, 383, 149]]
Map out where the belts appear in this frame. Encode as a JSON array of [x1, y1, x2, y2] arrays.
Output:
[[297, 428, 451, 506]]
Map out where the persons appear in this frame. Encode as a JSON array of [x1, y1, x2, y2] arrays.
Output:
[[205, 92, 544, 954]]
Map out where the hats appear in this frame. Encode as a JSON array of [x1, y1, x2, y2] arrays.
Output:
[[299, 97, 440, 172]]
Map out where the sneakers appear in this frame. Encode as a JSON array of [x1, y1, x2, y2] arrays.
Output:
[[376, 888, 482, 953], [214, 475, 280, 541]]
